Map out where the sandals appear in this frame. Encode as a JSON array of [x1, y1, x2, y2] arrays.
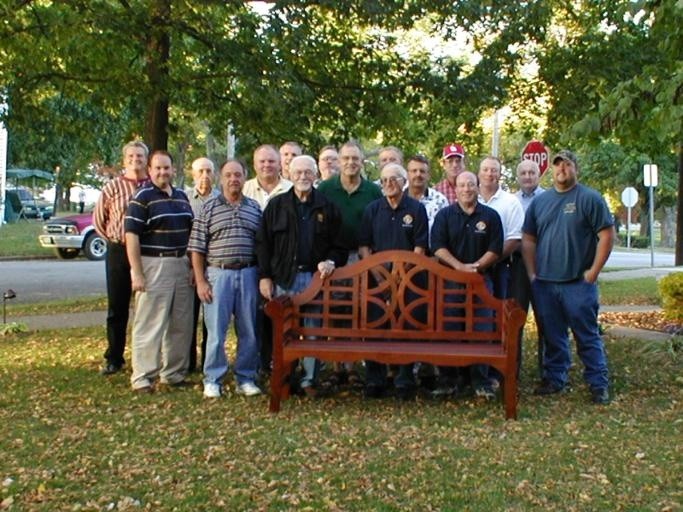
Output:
[[346, 370, 363, 390], [322, 371, 342, 389]]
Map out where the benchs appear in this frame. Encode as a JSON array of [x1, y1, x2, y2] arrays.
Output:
[[263, 250, 527, 422]]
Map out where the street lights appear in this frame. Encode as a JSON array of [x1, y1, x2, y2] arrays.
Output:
[[641, 156, 661, 270]]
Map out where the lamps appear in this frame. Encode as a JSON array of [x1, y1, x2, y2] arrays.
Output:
[[3, 290, 15, 322]]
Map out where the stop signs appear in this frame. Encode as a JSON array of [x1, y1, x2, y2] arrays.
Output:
[[520, 140, 552, 178]]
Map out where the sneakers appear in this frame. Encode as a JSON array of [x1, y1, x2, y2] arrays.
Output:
[[302, 385, 323, 395], [366, 381, 388, 397], [103, 357, 125, 374], [475, 380, 497, 400], [203, 382, 222, 398], [234, 382, 263, 397], [395, 384, 417, 400], [420, 378, 474, 399]]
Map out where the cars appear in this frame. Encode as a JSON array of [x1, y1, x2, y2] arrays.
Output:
[[5, 185, 56, 220], [36, 206, 110, 262]]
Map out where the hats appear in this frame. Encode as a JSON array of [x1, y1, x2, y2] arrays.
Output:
[[552, 150, 577, 165], [441, 145, 465, 161]]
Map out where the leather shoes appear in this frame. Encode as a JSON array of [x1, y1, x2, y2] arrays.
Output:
[[169, 380, 195, 387], [533, 380, 566, 396], [590, 387, 611, 405], [132, 386, 156, 395]]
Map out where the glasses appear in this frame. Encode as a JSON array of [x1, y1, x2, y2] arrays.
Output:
[[381, 176, 404, 185]]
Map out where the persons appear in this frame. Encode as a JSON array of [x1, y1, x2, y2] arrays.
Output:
[[91, 140, 614, 405], [79, 190, 86, 214]]
